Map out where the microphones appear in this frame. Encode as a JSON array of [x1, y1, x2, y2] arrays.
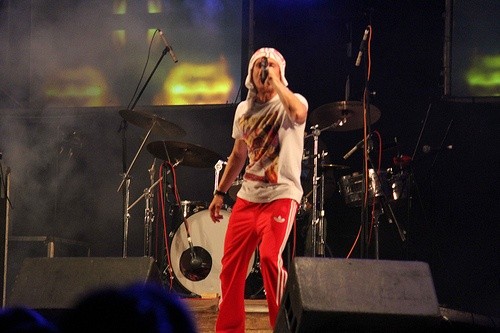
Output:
[[190, 256, 203, 269], [159, 30, 178, 64], [423, 144, 455, 153], [342, 135, 370, 159], [355, 29, 370, 66], [261, 56, 270, 84]]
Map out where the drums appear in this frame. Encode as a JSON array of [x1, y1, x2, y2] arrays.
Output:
[[169, 206, 264, 299], [169, 200, 208, 240], [338, 167, 410, 206], [222, 154, 250, 202]]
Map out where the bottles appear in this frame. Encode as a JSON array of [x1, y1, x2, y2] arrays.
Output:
[[46, 235, 55, 259]]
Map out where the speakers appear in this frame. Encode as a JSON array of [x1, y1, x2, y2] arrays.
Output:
[[5, 256, 162, 310], [272, 256, 439, 333]]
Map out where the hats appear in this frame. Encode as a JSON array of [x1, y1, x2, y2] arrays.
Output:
[[244, 47, 290, 90]]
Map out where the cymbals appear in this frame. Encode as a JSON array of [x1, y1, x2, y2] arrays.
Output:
[[144, 140, 225, 170], [118, 109, 187, 137], [301, 161, 343, 169], [309, 100, 382, 132]]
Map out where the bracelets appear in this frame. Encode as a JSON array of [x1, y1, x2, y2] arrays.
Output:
[[213, 189, 227, 197]]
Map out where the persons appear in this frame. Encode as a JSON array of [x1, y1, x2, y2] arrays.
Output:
[[0, 283, 202, 333], [208, 48, 308, 333]]
